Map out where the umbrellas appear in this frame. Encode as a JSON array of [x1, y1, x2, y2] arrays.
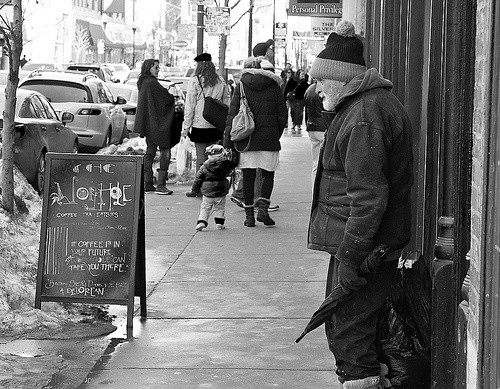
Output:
[[295, 244, 394, 344]]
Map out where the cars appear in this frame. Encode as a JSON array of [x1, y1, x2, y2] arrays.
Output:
[[0, 84, 78, 195], [0, 57, 243, 144]]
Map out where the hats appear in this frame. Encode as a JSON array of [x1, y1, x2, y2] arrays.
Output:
[[194, 53, 212, 62], [253, 39, 274, 57], [310, 20, 366, 82]]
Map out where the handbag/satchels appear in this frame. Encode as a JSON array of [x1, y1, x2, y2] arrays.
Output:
[[230, 104, 255, 140], [176, 135, 192, 175], [203, 96, 229, 131]]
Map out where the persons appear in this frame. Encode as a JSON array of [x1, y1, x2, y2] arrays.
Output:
[[134, 58, 175, 194], [182, 53, 230, 200], [224, 57, 288, 227], [306, 42, 414, 389], [192, 143, 240, 231], [226, 43, 285, 212], [304, 79, 328, 199], [280, 63, 317, 131]]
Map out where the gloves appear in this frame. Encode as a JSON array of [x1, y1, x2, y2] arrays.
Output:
[[338, 263, 367, 295]]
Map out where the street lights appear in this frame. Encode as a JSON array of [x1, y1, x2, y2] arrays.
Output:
[[132, 27, 136, 67]]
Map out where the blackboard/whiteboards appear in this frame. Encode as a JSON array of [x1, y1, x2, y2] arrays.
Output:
[[35, 152, 147, 304]]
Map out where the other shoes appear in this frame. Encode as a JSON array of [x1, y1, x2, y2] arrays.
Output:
[[216, 224, 225, 229], [186, 190, 202, 197], [291, 125, 302, 129], [230, 195, 257, 212], [253, 204, 280, 212], [196, 223, 205, 231]]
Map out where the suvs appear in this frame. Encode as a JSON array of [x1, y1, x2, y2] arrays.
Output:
[[17, 68, 128, 152]]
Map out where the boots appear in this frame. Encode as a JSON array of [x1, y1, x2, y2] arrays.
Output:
[[154, 170, 173, 195], [244, 204, 255, 227], [254, 196, 275, 225], [144, 171, 156, 193]]
